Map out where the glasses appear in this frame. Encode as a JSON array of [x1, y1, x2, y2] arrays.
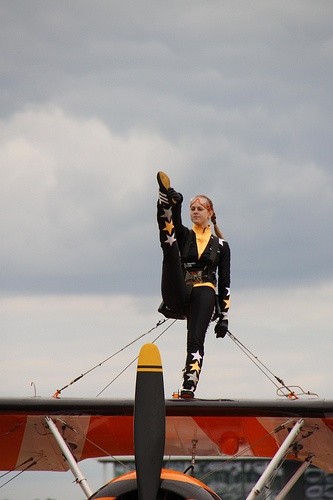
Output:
[[190, 197, 211, 211]]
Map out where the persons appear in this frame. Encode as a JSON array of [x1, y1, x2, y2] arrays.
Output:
[[156, 171, 231, 398]]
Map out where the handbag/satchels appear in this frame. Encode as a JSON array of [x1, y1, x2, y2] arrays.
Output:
[[157, 301, 184, 319]]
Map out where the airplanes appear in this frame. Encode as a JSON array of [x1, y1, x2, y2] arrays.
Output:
[[0, 344, 333, 500]]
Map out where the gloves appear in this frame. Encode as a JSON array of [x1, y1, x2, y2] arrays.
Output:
[[215, 320, 228, 338], [167, 187, 182, 202]]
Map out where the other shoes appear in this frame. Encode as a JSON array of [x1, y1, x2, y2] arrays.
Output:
[[181, 390, 194, 399], [157, 171, 178, 205]]
